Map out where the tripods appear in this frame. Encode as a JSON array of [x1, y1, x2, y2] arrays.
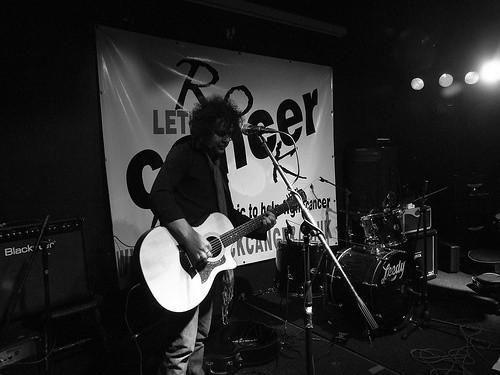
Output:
[[402, 180, 476, 340]]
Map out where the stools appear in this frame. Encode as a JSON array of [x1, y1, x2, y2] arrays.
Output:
[[40, 294, 114, 375]]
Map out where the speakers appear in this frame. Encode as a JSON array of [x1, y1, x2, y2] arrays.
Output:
[[403, 229, 438, 280], [0, 218, 89, 325]]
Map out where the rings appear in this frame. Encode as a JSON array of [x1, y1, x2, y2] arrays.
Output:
[[206, 250, 210, 254]]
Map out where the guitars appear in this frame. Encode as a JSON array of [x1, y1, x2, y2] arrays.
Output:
[[131, 188, 307, 312]]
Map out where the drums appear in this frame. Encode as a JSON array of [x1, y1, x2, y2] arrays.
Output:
[[271, 238, 326, 297], [327, 246, 417, 332], [360, 209, 407, 248]]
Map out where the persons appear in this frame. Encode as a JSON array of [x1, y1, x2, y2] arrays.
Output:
[[150, 95, 276, 375]]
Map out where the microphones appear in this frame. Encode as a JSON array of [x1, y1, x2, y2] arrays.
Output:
[[240, 123, 276, 135]]
